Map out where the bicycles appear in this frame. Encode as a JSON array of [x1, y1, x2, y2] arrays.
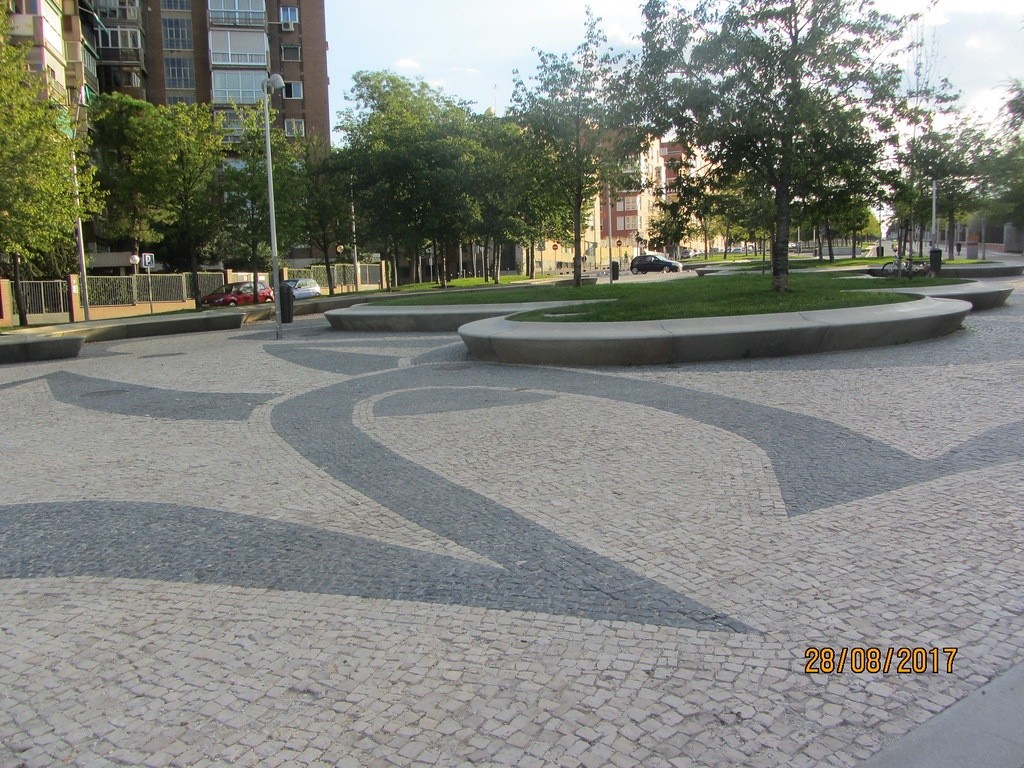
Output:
[[881, 249, 925, 278]]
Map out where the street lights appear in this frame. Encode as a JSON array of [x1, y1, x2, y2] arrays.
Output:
[[261, 73, 285, 339]]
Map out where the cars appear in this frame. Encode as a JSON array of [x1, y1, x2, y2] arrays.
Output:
[[272, 278, 321, 301], [201, 280, 274, 308], [680, 248, 704, 260], [630, 255, 683, 275]]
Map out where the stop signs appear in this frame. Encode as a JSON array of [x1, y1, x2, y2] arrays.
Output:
[[552, 244, 558, 250], [617, 240, 622, 246]]
